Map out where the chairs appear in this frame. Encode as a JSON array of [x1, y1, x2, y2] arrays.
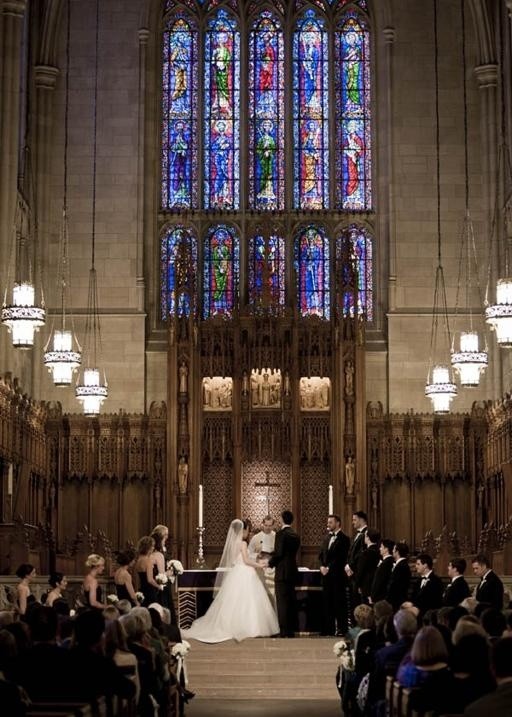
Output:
[[1, 612, 192, 717], [331, 605, 510, 714]]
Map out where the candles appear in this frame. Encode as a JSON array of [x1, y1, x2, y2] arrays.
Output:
[[327, 484, 333, 514], [197, 482, 204, 527]]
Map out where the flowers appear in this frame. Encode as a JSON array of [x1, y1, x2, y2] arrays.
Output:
[[107, 593, 119, 602], [166, 559, 184, 583], [136, 590, 145, 603]]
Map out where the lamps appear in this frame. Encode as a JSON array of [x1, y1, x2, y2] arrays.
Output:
[[422, 7, 460, 415], [480, 6, 511, 347], [74, 0, 110, 418], [447, 6, 489, 387], [0, 0, 48, 352], [40, 0, 83, 388]]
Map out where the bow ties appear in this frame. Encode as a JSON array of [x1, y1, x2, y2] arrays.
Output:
[[421, 577, 427, 580], [330, 532, 335, 537]]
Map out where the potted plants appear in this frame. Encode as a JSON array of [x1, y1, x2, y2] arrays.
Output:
[[155, 572, 168, 583]]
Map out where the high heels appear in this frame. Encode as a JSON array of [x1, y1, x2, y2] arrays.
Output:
[[181, 692, 195, 704]]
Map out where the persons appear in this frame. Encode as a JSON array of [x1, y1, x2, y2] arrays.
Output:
[[264, 511, 301, 638], [345, 457, 355, 494], [0, 523, 196, 717], [178, 457, 188, 493], [318, 515, 350, 637], [248, 514, 277, 609], [221, 520, 280, 644], [179, 361, 188, 392], [333, 511, 512, 717], [345, 361, 355, 395]]
